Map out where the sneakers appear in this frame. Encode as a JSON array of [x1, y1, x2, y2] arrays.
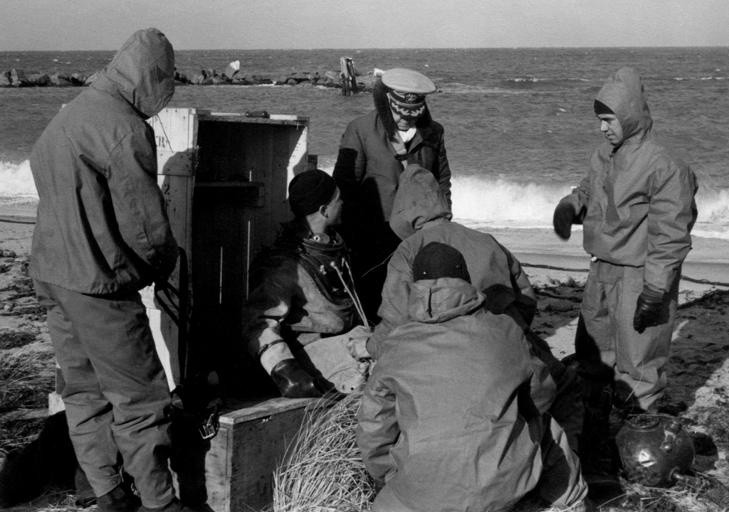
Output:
[[138, 498, 195, 511], [97, 483, 140, 510]]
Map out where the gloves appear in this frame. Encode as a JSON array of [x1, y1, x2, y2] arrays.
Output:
[[633, 294, 669, 334], [273, 358, 323, 399], [554, 203, 580, 240]]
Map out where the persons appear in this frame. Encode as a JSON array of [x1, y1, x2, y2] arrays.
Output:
[[359, 239, 590, 511], [380, 163, 576, 419], [328, 68, 452, 322], [238, 171, 375, 396], [553, 66, 698, 449], [28, 29, 205, 509]]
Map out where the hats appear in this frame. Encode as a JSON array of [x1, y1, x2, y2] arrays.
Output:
[[412, 242, 472, 282], [288, 170, 334, 215], [382, 68, 436, 120]]
